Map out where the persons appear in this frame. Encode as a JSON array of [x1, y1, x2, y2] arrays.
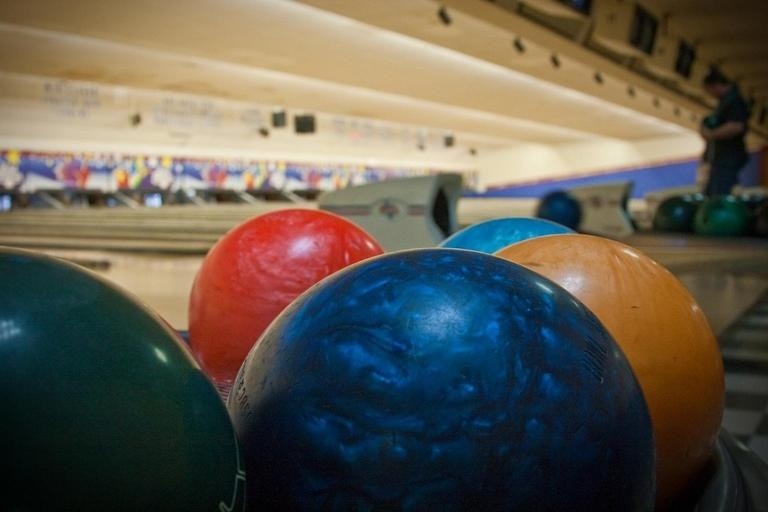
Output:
[[699, 68, 750, 197]]
